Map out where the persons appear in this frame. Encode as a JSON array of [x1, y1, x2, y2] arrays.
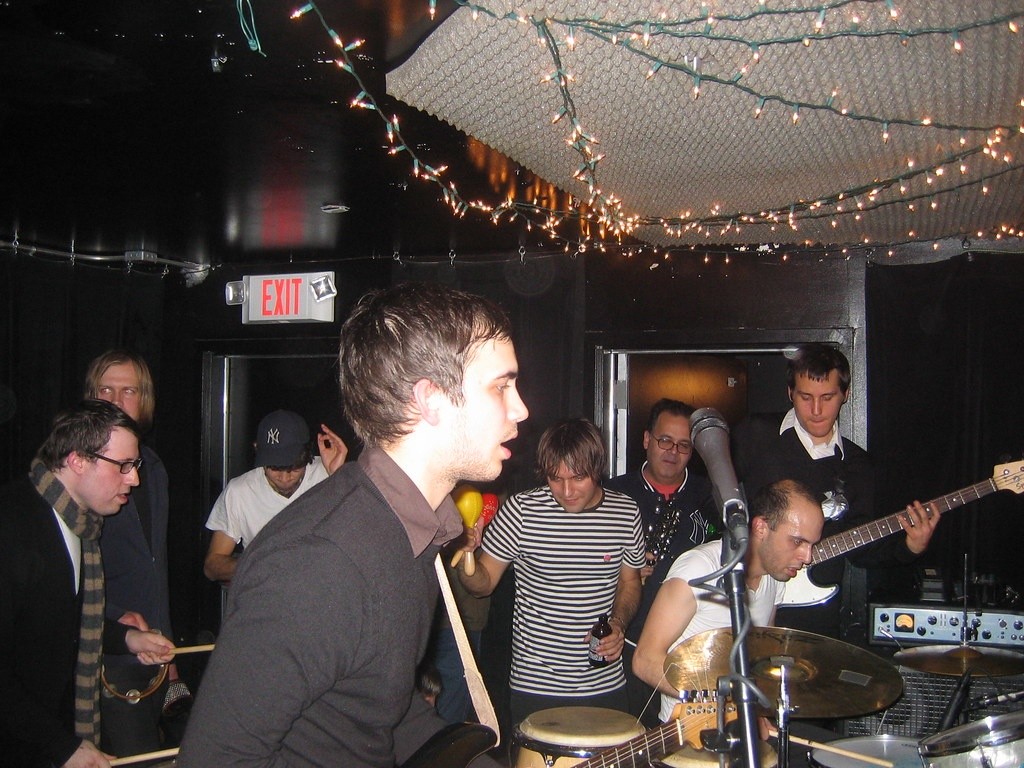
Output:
[[450, 417, 646, 729], [84, 349, 169, 758], [203, 411, 348, 580], [632, 479, 824, 724], [174, 283, 528, 768], [416, 542, 491, 729], [605, 398, 716, 726], [703, 343, 941, 640], [0, 397, 175, 768]]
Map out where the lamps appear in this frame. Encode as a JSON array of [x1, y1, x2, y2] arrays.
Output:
[[224, 270, 337, 324]]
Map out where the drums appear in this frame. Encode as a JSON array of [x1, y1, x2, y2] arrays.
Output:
[[660, 739, 778, 768], [514, 705, 648, 768], [917, 709, 1024, 768], [806, 734, 928, 768]]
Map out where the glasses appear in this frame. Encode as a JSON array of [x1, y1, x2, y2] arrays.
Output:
[[80, 449, 144, 473], [649, 432, 693, 455]]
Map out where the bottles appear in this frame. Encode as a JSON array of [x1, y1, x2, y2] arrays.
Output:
[[588, 614, 612, 668]]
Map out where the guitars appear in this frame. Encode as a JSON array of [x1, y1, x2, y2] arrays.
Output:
[[773, 459, 1024, 609], [398, 700, 739, 768]]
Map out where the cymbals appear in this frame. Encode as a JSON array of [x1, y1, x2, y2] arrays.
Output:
[[662, 625, 904, 719], [893, 643, 1024, 678], [100, 628, 169, 705]]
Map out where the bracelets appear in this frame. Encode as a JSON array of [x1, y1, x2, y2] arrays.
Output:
[[613, 617, 626, 633]]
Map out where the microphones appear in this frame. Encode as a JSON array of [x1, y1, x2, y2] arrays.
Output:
[[934, 668, 973, 734], [688, 408, 750, 544]]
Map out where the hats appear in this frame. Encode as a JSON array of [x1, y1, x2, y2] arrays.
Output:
[[256, 409, 309, 466]]
